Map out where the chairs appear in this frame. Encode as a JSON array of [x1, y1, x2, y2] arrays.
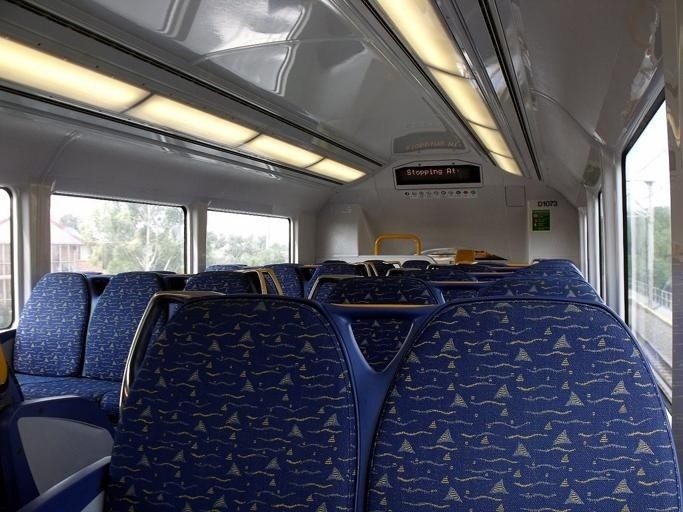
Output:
[[2, 256, 682, 506]]
